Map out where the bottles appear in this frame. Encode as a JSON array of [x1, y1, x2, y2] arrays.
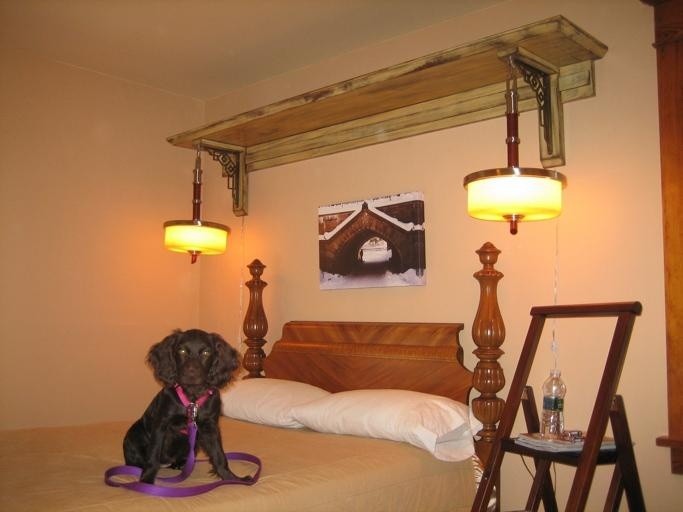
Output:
[[539, 369, 566, 439]]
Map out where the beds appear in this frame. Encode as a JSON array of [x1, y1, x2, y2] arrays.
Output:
[[0, 241, 505, 512]]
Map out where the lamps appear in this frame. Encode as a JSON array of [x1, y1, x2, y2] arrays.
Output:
[[161, 150, 231, 265], [461, 64, 567, 236]]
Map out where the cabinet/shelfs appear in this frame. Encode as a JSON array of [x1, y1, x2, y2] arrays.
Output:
[[468, 300, 648, 511]]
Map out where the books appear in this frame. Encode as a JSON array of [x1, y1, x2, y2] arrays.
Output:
[[512, 426, 633, 454]]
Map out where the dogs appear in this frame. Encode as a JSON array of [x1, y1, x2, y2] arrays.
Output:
[[122, 328, 254, 485]]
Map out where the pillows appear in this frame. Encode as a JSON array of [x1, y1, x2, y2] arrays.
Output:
[[288, 388, 482, 462], [219, 379, 332, 430]]
